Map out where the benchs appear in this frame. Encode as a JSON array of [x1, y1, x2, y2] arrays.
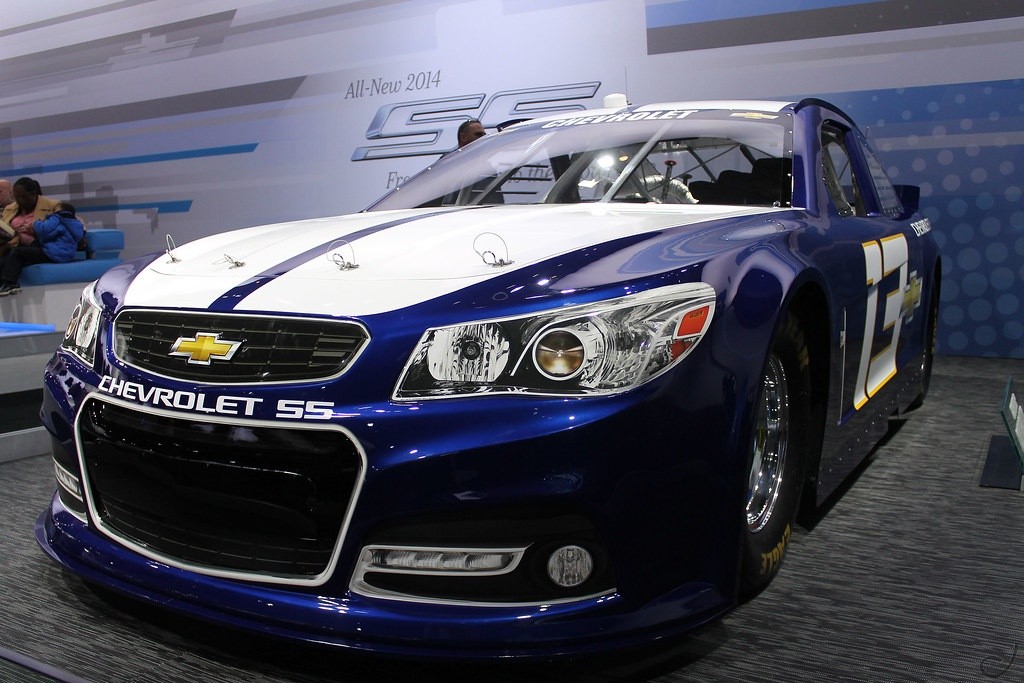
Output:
[[16, 229, 127, 284]]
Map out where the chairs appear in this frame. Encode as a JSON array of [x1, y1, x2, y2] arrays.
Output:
[[707, 170, 753, 203], [751, 157, 793, 207]]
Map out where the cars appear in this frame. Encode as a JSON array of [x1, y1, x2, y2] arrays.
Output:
[[33, 93, 943, 659]]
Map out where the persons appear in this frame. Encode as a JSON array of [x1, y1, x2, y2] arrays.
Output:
[[436, 118, 508, 208], [0, 176, 61, 272], [0, 202, 84, 296], [0, 180, 17, 220]]
